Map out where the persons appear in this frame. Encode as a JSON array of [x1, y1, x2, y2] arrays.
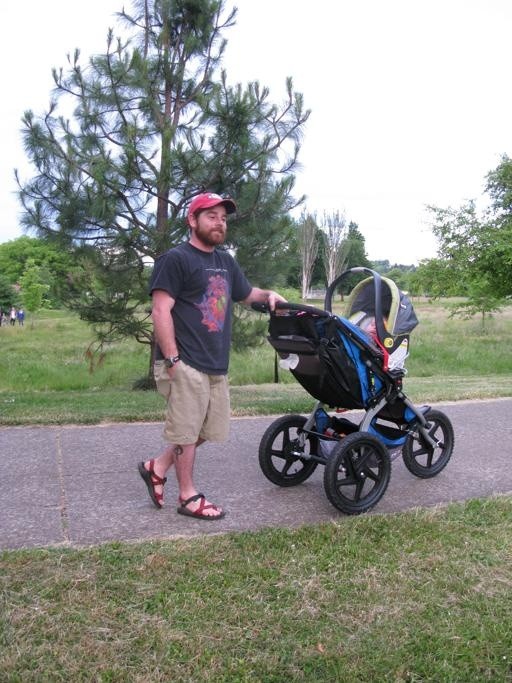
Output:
[[0, 306, 25, 327], [365, 316, 388, 336], [138, 192, 289, 520]]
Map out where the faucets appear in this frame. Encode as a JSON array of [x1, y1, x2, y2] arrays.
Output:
[[138, 459, 167, 507], [177, 492, 226, 519]]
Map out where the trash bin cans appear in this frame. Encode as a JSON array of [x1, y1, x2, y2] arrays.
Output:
[[164, 356, 180, 368]]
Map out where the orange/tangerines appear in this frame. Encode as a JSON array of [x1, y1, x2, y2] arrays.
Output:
[[250, 266, 455, 516]]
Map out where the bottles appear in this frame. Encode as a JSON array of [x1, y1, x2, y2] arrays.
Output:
[[187, 192, 237, 220]]
